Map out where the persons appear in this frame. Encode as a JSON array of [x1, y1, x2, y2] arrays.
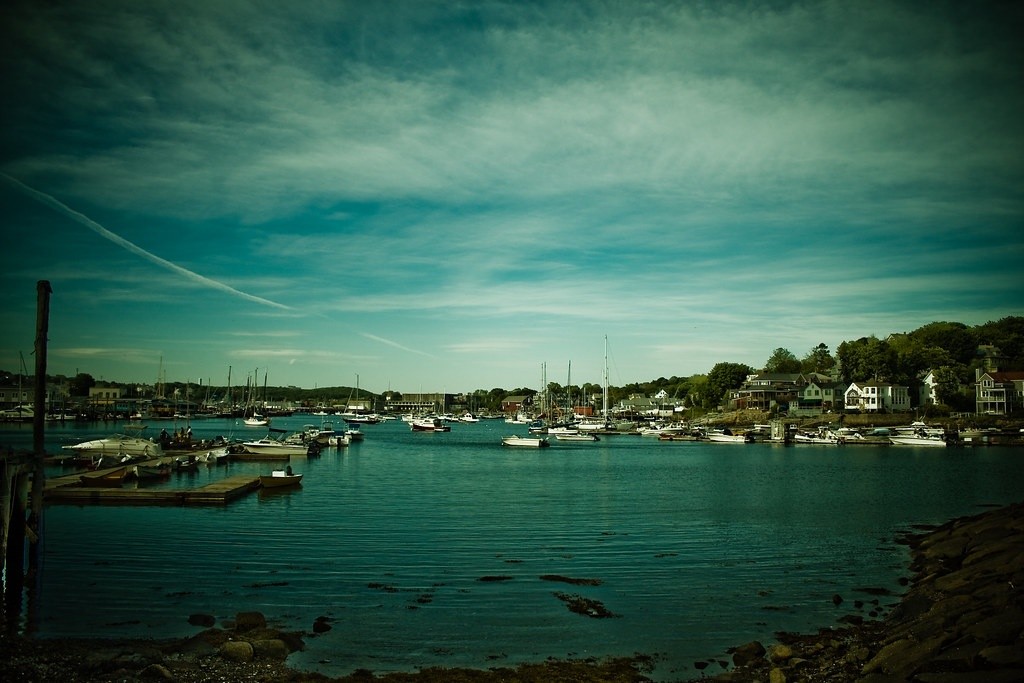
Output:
[[161, 426, 192, 441], [914, 428, 926, 438]]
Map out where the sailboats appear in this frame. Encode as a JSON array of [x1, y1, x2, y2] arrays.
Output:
[[0, 352, 294, 427], [407, 384, 424, 426], [528, 334, 634, 434], [340, 374, 381, 425]]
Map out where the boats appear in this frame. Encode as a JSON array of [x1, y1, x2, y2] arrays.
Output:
[[132, 461, 173, 479], [61, 426, 167, 461], [197, 448, 230, 463], [258, 466, 303, 488], [636, 413, 998, 446], [499, 435, 551, 448], [177, 455, 201, 471], [78, 466, 128, 487], [382, 412, 479, 425], [285, 420, 365, 456], [554, 433, 600, 442], [412, 418, 452, 432], [314, 411, 328, 416], [242, 434, 309, 455], [335, 410, 359, 416]]
